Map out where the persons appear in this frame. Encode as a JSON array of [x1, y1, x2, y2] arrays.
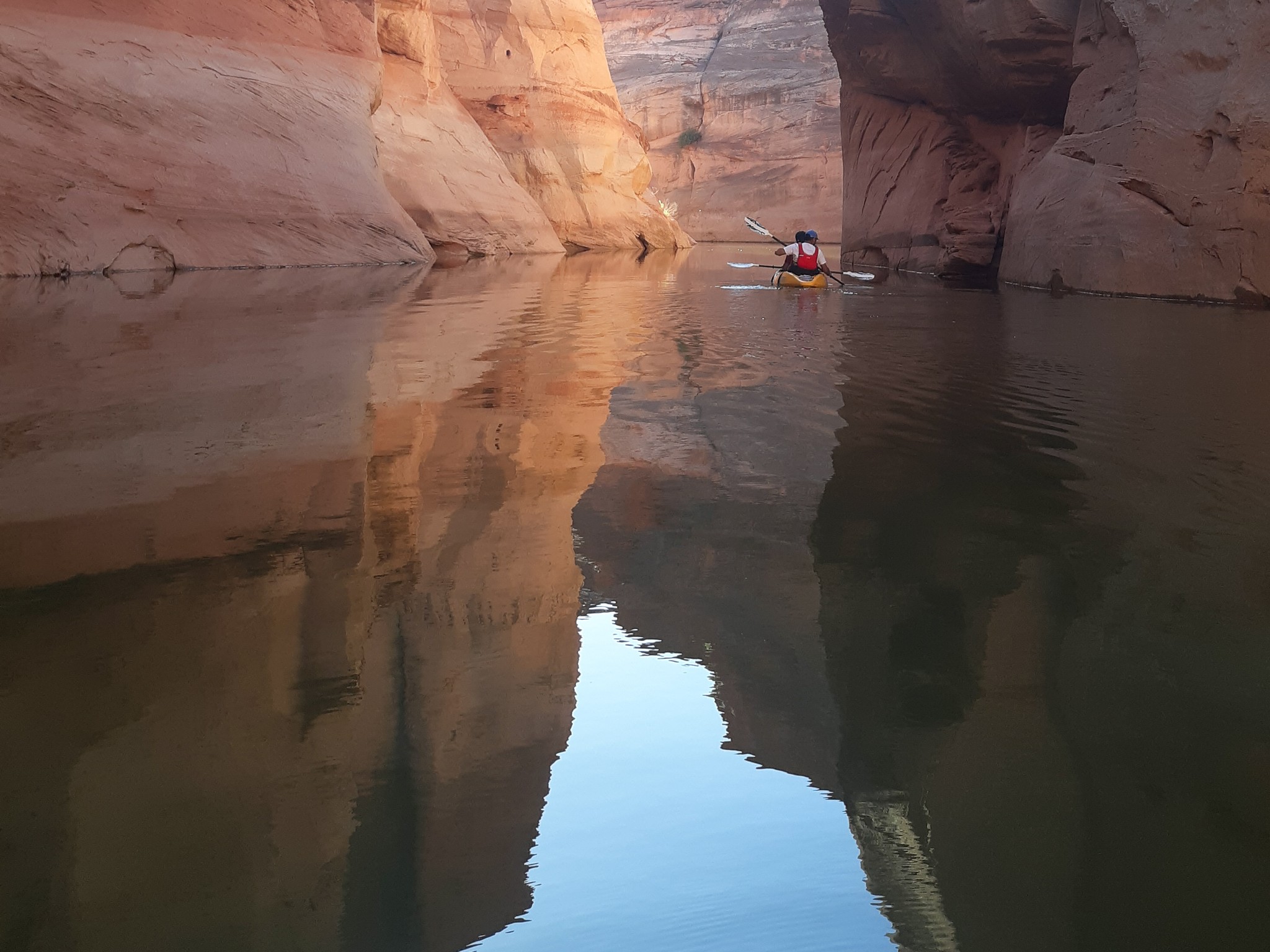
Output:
[[775, 230, 832, 277]]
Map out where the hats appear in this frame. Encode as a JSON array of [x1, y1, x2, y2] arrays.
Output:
[[805, 230, 820, 240], [795, 230, 806, 242]]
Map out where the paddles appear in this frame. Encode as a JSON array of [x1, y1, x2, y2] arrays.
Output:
[[727, 262, 875, 280], [744, 216, 845, 285]]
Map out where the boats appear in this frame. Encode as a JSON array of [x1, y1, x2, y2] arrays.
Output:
[[771, 269, 829, 289]]
